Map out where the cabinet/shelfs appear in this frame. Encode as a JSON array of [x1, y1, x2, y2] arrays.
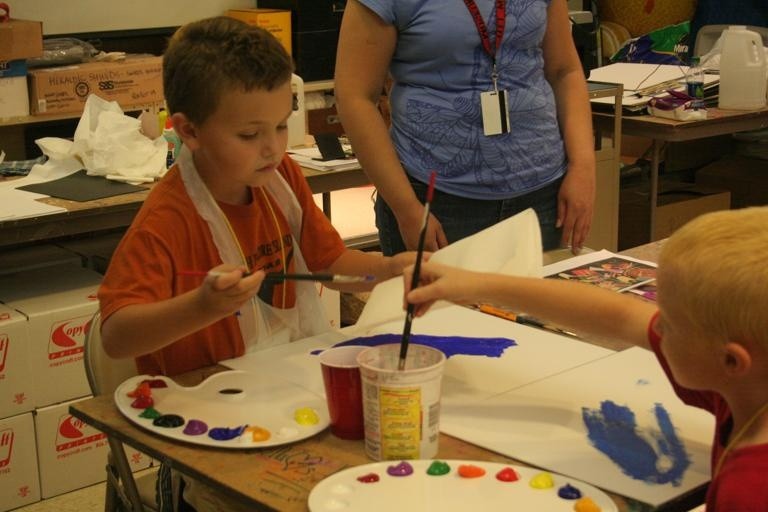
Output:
[[578, 77, 624, 255], [284, 68, 338, 150]]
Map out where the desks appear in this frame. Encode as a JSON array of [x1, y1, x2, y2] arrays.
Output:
[[63, 236, 733, 510], [583, 101, 766, 250], [0, 104, 169, 181], [1, 131, 375, 240]]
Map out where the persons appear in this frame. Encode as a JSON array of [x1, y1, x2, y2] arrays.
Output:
[[402, 207, 767, 512], [99, 18, 435, 512], [333, 2, 595, 255]]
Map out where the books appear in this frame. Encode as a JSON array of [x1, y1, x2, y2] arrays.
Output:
[[289, 153, 359, 172], [585, 63, 721, 117]]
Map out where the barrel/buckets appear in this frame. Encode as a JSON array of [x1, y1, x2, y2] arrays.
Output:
[[718, 24, 767, 111], [287, 72, 309, 149]]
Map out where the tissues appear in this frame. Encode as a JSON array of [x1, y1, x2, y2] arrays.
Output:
[[647, 88, 708, 122]]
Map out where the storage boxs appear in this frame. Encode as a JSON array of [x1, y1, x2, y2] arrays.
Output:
[[226, 5, 293, 54], [618, 179, 732, 252], [0, 18, 169, 118], [1, 235, 169, 512]]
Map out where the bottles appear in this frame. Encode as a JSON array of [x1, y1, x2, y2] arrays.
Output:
[[159, 107, 180, 164], [682, 53, 707, 110]]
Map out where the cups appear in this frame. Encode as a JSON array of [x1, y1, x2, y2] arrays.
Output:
[[322, 344, 368, 440]]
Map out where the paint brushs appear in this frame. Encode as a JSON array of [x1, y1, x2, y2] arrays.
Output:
[[174, 269, 375, 283], [399, 169, 437, 370], [479, 304, 578, 337]]
